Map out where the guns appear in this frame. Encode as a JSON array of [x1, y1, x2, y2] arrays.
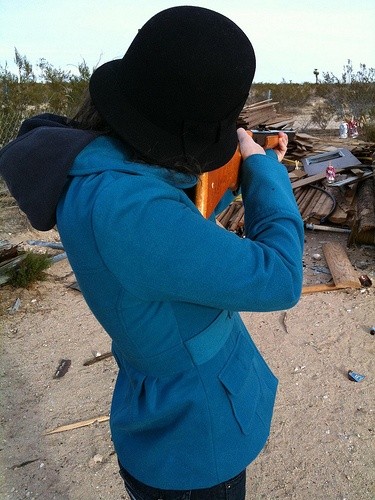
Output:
[[192, 127, 297, 218]]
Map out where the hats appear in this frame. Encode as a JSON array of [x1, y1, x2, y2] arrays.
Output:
[[88, 6, 256, 176]]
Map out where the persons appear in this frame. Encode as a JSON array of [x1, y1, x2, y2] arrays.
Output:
[[0, 5, 304, 500]]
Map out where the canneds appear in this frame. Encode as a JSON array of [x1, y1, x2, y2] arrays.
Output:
[[348, 121, 359, 139], [339, 122, 348, 139], [325, 166, 335, 184]]
